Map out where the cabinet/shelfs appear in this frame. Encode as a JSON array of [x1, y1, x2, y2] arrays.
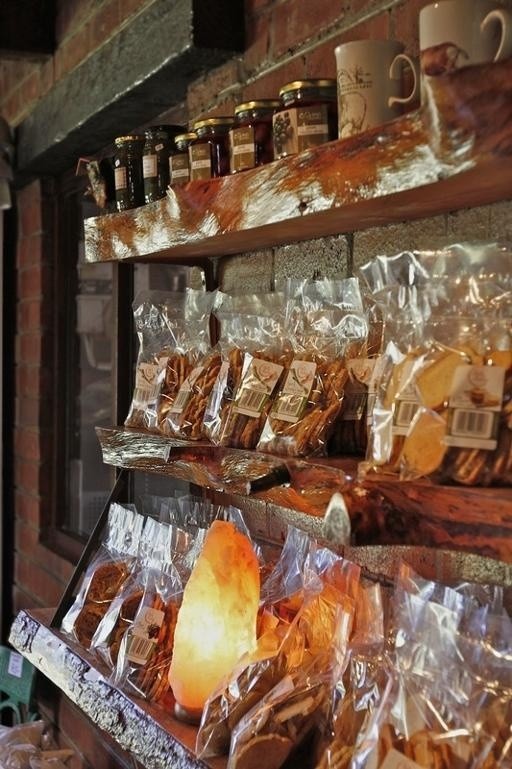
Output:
[[8, 91, 512, 769]]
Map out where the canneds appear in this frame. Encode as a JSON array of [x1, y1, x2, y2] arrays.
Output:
[[114, 78, 338, 212]]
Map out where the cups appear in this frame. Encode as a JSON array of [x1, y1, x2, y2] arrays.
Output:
[[335, 0, 512, 141]]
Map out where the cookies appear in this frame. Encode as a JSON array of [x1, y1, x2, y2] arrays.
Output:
[[75, 562, 512, 769], [158, 335, 512, 487]]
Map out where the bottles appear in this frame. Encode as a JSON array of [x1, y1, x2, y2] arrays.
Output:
[[115, 78, 336, 212]]
